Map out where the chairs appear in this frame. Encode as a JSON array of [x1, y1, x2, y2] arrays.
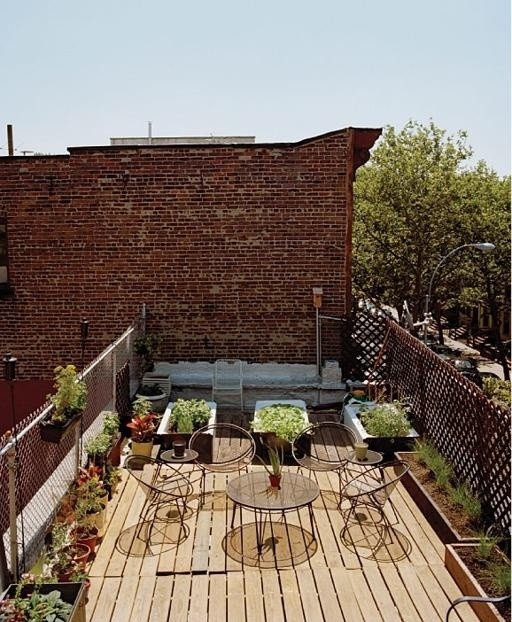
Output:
[[123, 423, 411, 559], [210, 358, 244, 410]]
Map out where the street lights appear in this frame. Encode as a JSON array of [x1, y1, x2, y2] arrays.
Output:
[[424, 240, 496, 346]]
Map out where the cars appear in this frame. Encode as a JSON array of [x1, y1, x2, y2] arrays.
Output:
[[355, 321, 483, 391]]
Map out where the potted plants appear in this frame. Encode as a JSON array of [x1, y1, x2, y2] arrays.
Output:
[[136, 383, 165, 408], [0, 367, 120, 622], [254, 399, 310, 464], [156, 401, 215, 461], [343, 400, 417, 449], [130, 413, 154, 457], [342, 451, 511, 622]]
